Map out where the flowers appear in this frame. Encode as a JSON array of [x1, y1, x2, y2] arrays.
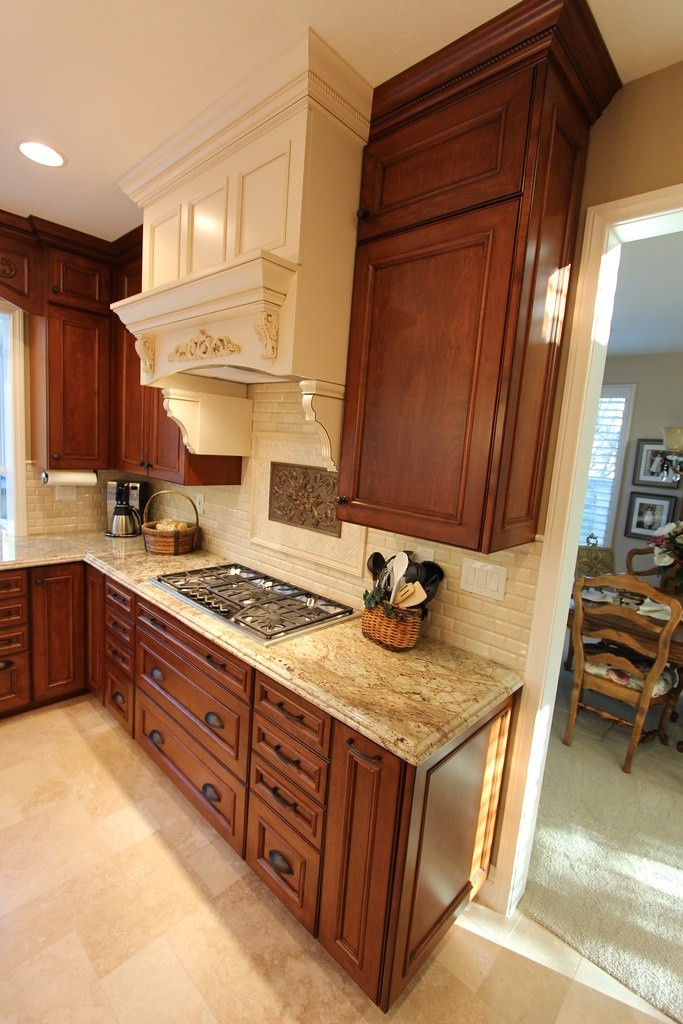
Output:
[[646, 518, 683, 577]]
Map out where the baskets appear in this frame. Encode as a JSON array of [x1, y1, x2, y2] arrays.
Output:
[[361, 599, 423, 652]]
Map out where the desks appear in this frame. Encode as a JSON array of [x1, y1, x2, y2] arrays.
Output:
[[562, 586, 683, 673]]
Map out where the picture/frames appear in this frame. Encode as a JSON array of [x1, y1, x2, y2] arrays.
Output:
[[623, 492, 676, 539], [631, 439, 680, 488]]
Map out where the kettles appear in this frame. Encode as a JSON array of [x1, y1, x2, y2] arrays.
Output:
[[111, 504, 142, 536]]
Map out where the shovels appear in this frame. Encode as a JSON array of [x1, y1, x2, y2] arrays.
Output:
[[397, 580, 428, 607], [389, 551, 409, 605]]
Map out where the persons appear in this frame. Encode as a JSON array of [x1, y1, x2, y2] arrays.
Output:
[[650, 452, 664, 475], [643, 506, 654, 528]]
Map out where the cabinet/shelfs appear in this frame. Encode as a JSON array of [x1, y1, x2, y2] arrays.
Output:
[[334, 35, 590, 558], [0, 561, 86, 714], [104, 579, 520, 1007], [42, 248, 242, 486]]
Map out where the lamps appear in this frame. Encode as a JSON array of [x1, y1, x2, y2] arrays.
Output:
[[662, 425, 683, 477]]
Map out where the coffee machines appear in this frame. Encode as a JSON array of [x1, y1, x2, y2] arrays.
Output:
[[106, 481, 148, 536]]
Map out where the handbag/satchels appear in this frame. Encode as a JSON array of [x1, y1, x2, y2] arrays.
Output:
[[141, 490, 198, 556]]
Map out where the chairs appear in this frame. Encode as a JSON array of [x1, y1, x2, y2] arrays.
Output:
[[563, 561, 682, 772]]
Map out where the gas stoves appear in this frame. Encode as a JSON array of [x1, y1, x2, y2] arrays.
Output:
[[147, 562, 364, 647]]
